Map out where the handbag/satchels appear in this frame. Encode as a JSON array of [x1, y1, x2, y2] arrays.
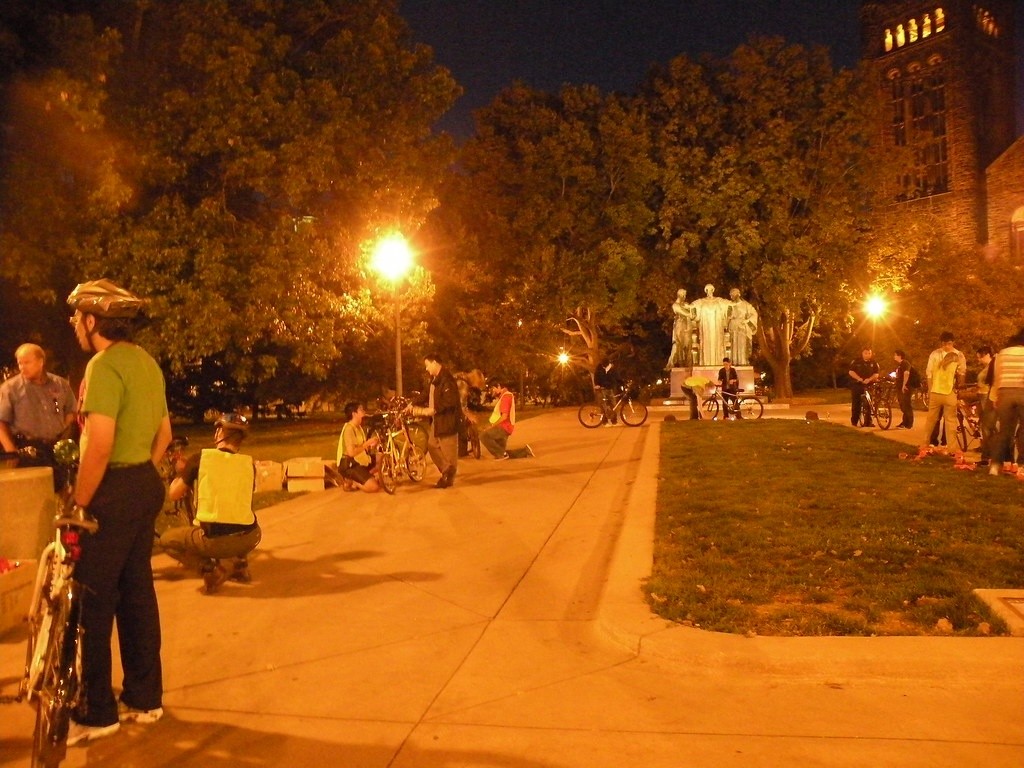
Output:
[[902, 360, 921, 388]]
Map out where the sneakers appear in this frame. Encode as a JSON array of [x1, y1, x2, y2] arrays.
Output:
[[66, 717, 121, 749], [115, 697, 164, 723], [231, 562, 251, 582], [197, 564, 228, 595]]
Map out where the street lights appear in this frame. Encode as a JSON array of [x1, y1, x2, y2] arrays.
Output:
[[863, 294, 890, 351], [369, 238, 416, 398]]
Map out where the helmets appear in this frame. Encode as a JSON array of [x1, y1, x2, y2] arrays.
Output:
[[67, 276, 150, 318], [214, 413, 250, 438]]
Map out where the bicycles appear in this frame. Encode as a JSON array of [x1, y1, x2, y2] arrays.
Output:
[[910, 378, 929, 410], [955, 383, 1000, 453], [461, 414, 482, 460], [859, 376, 897, 430], [700, 385, 763, 421], [16, 439, 98, 768], [360, 390, 430, 495], [577, 385, 648, 428], [152, 435, 198, 540]]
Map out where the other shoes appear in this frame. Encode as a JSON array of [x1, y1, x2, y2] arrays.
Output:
[[434, 474, 454, 489], [525, 445, 535, 457], [896, 424, 913, 430], [494, 453, 509, 461], [1017, 467, 1024, 479], [988, 464, 997, 475], [974, 461, 990, 466], [343, 476, 353, 492]]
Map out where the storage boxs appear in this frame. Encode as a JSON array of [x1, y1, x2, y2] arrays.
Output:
[[0, 559, 39, 634], [255, 457, 337, 494]]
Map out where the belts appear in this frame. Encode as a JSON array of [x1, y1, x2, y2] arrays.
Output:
[[227, 524, 257, 536]]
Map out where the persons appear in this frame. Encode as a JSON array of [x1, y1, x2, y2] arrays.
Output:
[[159, 413, 262, 594], [847, 330, 1024, 483], [717, 357, 743, 421], [592, 358, 624, 426], [0, 342, 79, 508], [55, 279, 164, 746], [668, 285, 759, 366], [337, 351, 536, 492], [681, 376, 715, 420]]
